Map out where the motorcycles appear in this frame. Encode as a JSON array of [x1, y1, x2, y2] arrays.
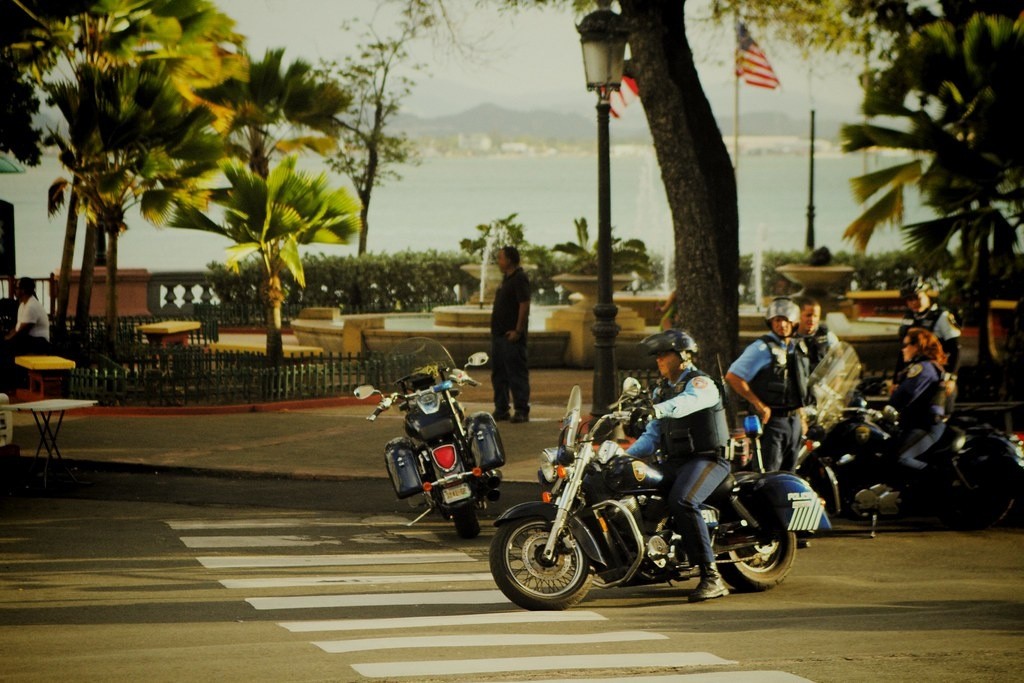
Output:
[[798, 375, 1024, 538], [354, 337, 506, 540], [487, 377, 833, 612]]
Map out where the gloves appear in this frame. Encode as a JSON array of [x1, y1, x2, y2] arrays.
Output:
[[630, 407, 656, 427]]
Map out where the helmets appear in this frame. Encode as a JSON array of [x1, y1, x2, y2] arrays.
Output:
[[899, 282, 930, 303], [765, 297, 802, 327], [638, 330, 698, 356]]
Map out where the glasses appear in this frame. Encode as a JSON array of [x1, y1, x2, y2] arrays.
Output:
[[15, 286, 23, 290], [901, 342, 912, 348]]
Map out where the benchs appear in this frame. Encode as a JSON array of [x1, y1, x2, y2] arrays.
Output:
[[136, 321, 202, 349], [13, 356, 76, 401]]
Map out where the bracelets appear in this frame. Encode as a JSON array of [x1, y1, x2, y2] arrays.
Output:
[[950, 373, 958, 381], [515, 330, 522, 335]]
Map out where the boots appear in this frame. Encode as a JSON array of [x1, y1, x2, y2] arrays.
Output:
[[688, 562, 729, 602]]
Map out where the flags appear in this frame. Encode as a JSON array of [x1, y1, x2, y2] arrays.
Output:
[[735, 19, 780, 90], [601, 60, 640, 117]]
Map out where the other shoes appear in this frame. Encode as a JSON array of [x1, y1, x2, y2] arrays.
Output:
[[511, 413, 528, 422], [796, 539, 811, 548], [493, 412, 510, 420]]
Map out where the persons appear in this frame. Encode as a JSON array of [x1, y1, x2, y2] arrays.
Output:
[[886, 277, 961, 491], [0, 277, 50, 394], [655, 289, 678, 332], [726, 297, 844, 549], [624, 331, 735, 601], [490, 245, 530, 423]]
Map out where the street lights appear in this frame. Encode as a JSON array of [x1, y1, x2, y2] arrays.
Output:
[[573, 0, 637, 429]]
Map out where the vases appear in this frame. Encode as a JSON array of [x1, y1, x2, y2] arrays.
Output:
[[774, 262, 855, 294]]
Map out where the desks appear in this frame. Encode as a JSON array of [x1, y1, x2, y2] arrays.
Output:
[[1, 399, 98, 491]]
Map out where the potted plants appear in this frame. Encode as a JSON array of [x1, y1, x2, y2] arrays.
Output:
[[458, 211, 539, 287], [546, 218, 652, 299]]
